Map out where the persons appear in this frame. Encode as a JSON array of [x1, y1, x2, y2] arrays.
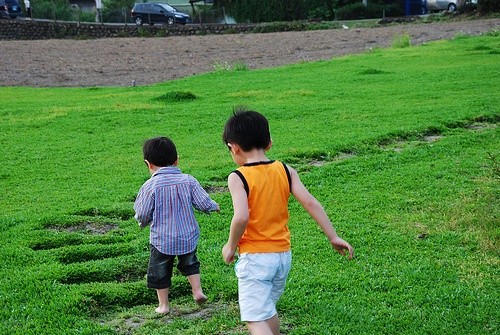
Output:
[[24, 0, 31, 20], [221, 105, 354, 335], [133, 137, 220, 313]]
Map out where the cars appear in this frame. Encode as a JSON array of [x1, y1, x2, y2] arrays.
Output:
[[0, 0, 23, 20], [421, 0, 478, 15]]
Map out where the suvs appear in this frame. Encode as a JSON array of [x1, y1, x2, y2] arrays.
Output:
[[130, 2, 193, 26]]
